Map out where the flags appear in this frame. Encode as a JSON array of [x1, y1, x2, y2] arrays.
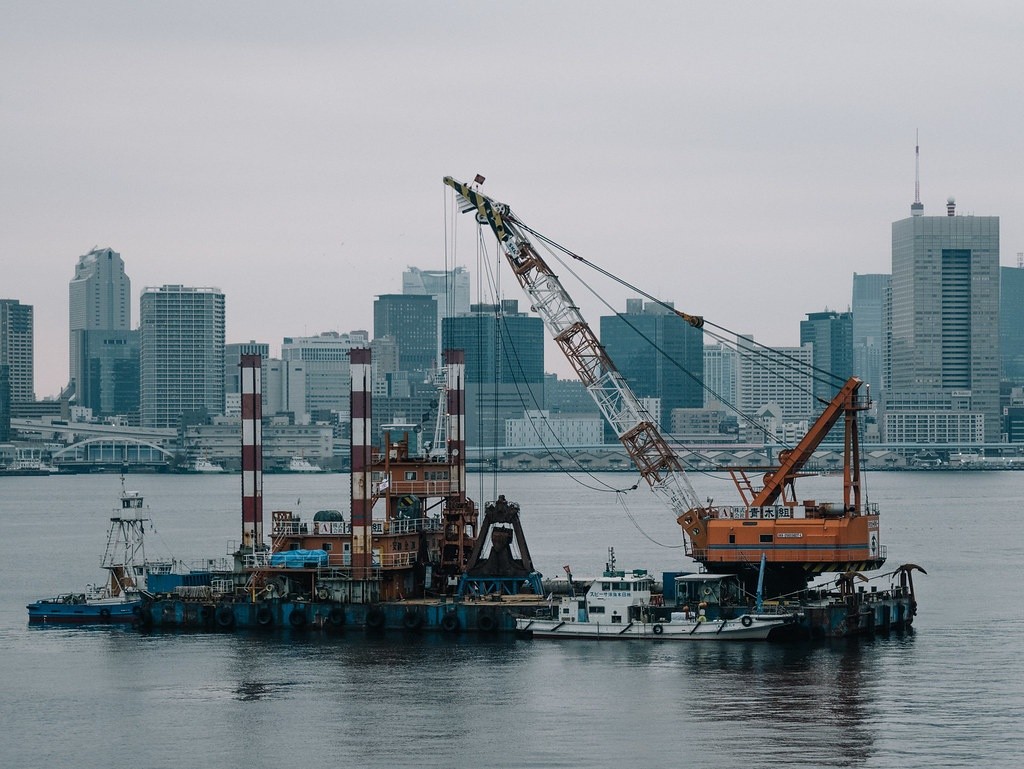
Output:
[[474, 174, 485, 185]]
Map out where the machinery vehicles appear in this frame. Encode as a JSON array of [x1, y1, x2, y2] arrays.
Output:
[[439, 171, 889, 584]]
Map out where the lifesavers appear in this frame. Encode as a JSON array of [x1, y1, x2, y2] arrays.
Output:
[[657, 594, 662, 606], [403, 611, 424, 631], [328, 608, 346, 628], [653, 624, 663, 635], [289, 609, 306, 628], [133, 606, 141, 616], [217, 609, 234, 628], [742, 616, 752, 627], [704, 587, 712, 595], [808, 625, 825, 642], [479, 614, 496, 633], [200, 609, 209, 619], [652, 596, 657, 606], [100, 608, 111, 619], [366, 607, 385, 630], [257, 608, 273, 627], [442, 614, 460, 635]]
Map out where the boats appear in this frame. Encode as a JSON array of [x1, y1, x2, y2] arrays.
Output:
[[148, 346, 928, 649], [22, 463, 160, 621], [510, 575, 805, 642]]
